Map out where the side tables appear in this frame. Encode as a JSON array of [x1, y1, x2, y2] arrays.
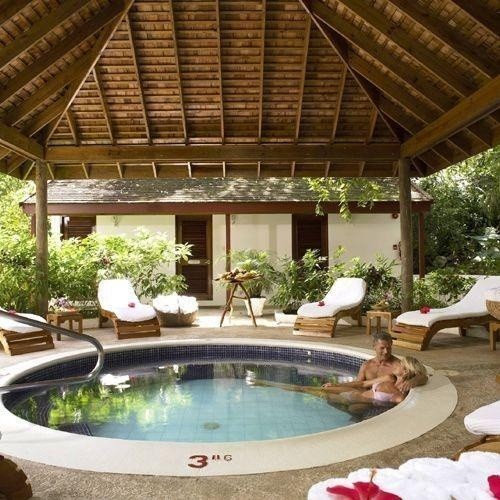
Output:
[[46, 312, 83, 341]]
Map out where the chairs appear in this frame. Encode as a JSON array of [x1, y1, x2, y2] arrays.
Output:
[[292, 276, 500, 351], [97, 278, 161, 341], [0, 306, 54, 357]]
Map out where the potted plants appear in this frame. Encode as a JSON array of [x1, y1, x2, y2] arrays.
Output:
[[213, 247, 287, 323]]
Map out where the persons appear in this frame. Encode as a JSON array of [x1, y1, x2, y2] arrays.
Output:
[[249, 356, 425, 405], [328, 331, 429, 423]]
